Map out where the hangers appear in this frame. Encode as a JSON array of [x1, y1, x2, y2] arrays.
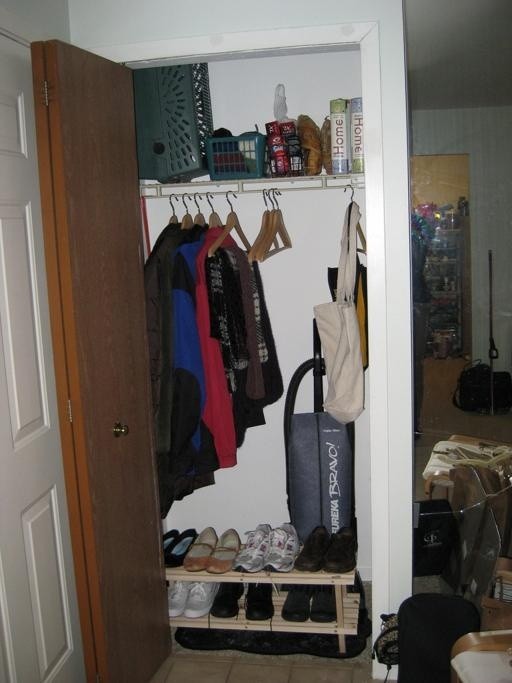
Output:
[[138, 184, 368, 266]]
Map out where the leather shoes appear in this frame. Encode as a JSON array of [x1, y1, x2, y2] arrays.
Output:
[[294, 525, 330, 572], [161, 527, 240, 574], [323, 524, 358, 573]]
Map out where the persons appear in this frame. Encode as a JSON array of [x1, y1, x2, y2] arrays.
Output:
[[412, 211, 443, 441]]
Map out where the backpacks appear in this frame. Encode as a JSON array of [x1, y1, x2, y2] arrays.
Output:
[[452, 359, 494, 414]]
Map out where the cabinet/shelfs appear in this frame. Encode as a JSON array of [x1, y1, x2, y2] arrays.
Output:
[[413, 209, 472, 359], [165, 560, 362, 660]]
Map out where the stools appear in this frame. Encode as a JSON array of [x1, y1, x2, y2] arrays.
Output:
[[424, 435, 512, 498]]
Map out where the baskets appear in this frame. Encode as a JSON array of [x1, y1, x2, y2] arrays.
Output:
[[203, 133, 267, 180], [131, 62, 211, 184]]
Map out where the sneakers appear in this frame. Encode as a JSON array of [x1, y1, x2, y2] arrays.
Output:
[[310, 585, 337, 623], [281, 585, 310, 624], [167, 580, 190, 618], [211, 582, 243, 620], [245, 580, 274, 621], [232, 522, 302, 573], [184, 578, 219, 619]]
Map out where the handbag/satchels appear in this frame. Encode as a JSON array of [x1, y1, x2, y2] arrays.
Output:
[[314, 300, 366, 427], [414, 483, 456, 579], [461, 576, 512, 632], [282, 359, 355, 541]]
